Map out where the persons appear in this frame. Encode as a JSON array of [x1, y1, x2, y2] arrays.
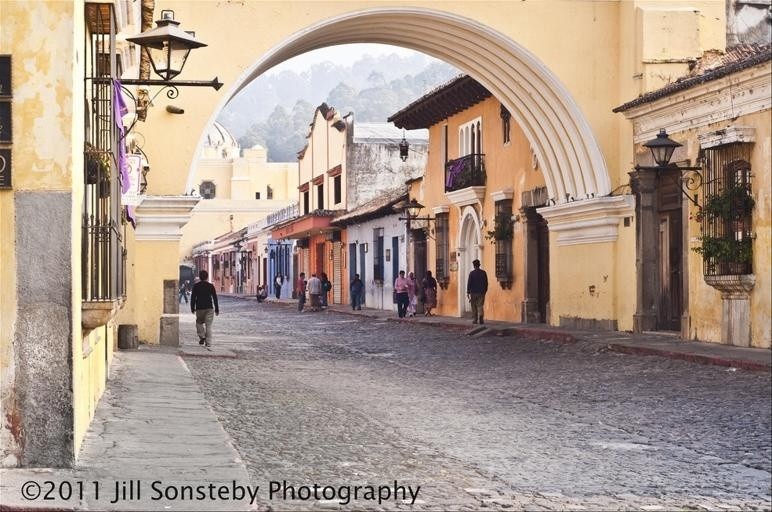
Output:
[[394, 270, 411, 319], [179, 280, 188, 304], [184, 280, 190, 296], [421, 270, 437, 316], [406, 271, 420, 317], [190, 269, 220, 351], [258, 286, 266, 303], [307, 273, 322, 311], [350, 274, 363, 310], [466, 259, 488, 325], [274, 271, 284, 300], [296, 271, 307, 313], [319, 271, 330, 309]]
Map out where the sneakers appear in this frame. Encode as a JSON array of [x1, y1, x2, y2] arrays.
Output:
[[199, 336, 211, 347], [472, 315, 484, 324]]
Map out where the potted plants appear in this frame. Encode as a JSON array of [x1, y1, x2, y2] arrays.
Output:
[[719, 238, 753, 274], [694, 233, 729, 275]]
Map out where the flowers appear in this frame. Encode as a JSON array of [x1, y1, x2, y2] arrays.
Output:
[[689, 185, 755, 222]]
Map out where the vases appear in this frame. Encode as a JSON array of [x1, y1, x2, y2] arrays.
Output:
[[728, 215, 752, 232]]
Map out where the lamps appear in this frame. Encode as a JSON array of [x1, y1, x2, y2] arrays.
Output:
[[94, 10, 224, 149], [397, 198, 437, 241], [398, 127, 410, 161], [632, 127, 704, 211]]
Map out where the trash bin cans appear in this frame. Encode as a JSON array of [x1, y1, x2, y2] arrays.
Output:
[[117, 324, 138, 349]]
